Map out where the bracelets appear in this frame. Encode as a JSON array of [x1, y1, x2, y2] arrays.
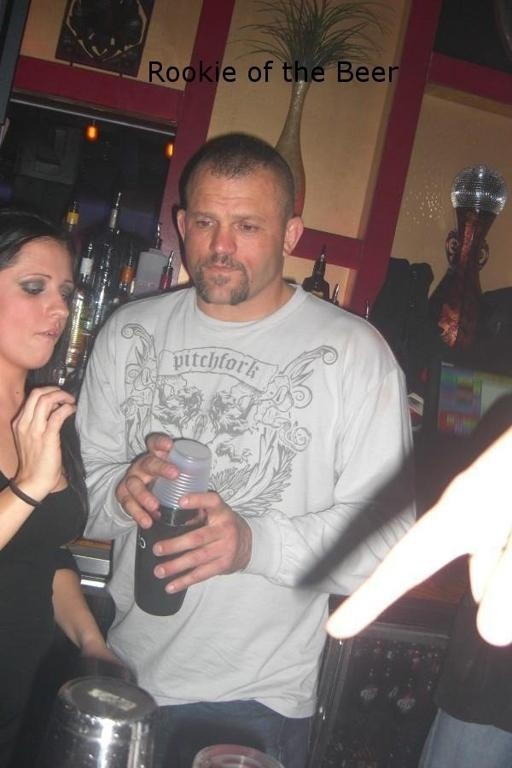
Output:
[[7, 480, 40, 508]]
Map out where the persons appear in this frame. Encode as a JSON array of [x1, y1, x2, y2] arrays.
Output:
[[74, 133, 415, 768], [0, 213, 128, 768], [323, 423, 512, 649], [412, 394, 512, 768]]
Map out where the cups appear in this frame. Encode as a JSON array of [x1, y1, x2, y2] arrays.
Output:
[[45, 677, 283, 768], [126, 438, 212, 617]]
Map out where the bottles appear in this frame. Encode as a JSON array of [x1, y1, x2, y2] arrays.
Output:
[[59, 190, 174, 379], [302, 245, 330, 300]]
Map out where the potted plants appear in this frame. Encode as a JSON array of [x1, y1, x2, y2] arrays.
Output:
[[226, 1, 398, 221]]
